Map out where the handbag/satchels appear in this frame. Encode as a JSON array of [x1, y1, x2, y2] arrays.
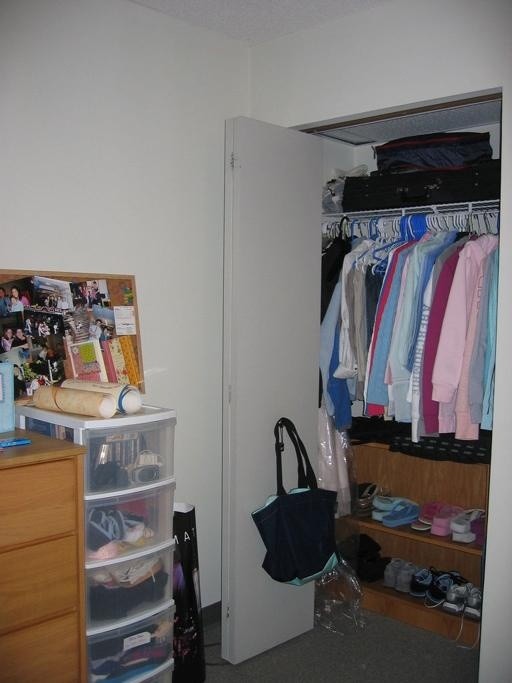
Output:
[[251, 417, 343, 587]]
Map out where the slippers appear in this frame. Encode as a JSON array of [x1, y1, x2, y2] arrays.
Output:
[[351, 480, 487, 549]]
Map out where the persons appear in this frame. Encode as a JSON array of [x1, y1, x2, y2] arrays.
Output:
[[0, 274, 113, 402]]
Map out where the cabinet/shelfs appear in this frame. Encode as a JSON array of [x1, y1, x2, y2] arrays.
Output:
[[323, 437, 487, 649], [0, 427, 87, 683]]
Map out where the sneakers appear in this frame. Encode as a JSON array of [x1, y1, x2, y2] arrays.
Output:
[[382, 558, 416, 592], [442, 581, 483, 622], [410, 566, 461, 609]]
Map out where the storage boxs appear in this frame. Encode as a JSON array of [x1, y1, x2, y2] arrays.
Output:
[[129, 659, 177, 683], [76, 478, 177, 568], [83, 601, 174, 682], [19, 400, 178, 502], [78, 540, 178, 627]]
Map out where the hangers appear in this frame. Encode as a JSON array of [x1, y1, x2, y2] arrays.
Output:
[[323, 208, 500, 245]]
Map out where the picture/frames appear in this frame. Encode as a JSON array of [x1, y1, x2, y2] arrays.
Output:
[[0, 361, 17, 433]]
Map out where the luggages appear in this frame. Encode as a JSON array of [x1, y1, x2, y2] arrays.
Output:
[[368, 131, 493, 177], [341, 159, 501, 214]]
